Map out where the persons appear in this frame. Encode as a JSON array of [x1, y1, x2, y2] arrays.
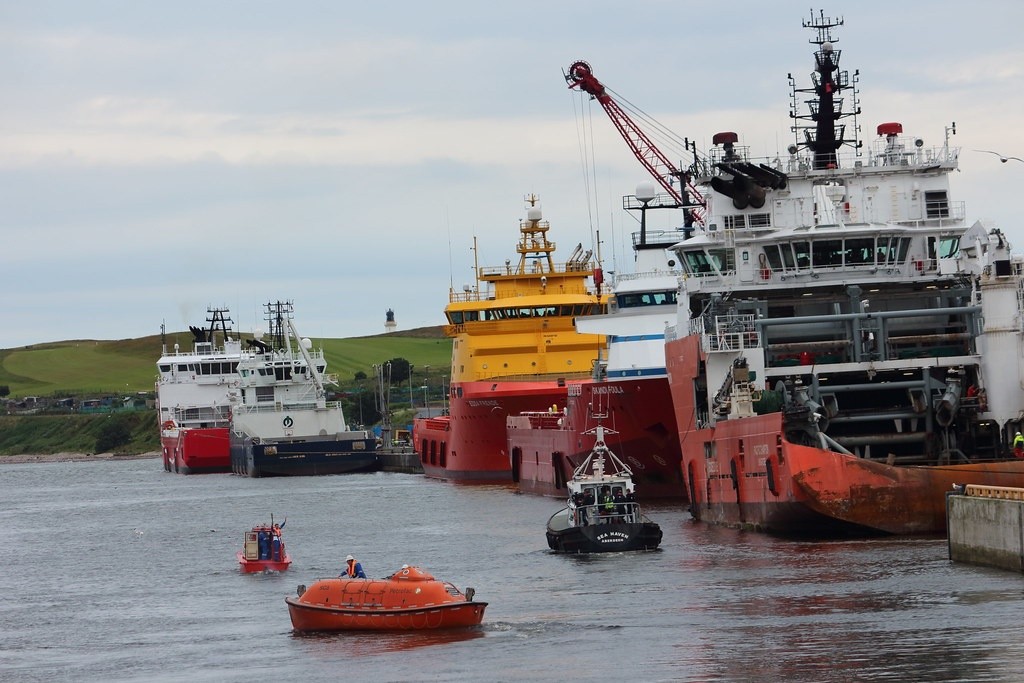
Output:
[[381, 563, 409, 580], [967, 384, 977, 404], [346, 422, 359, 431], [1013, 432, 1024, 458], [568, 489, 639, 524], [339, 555, 366, 578], [259, 518, 287, 561]]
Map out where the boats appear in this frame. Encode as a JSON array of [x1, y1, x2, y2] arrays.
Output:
[[236, 513, 292, 574], [545, 378, 663, 553], [155, 303, 267, 474], [507, 182, 705, 501], [411, 191, 618, 486], [562, 5, 1024, 536], [283, 564, 488, 630], [226, 298, 380, 476]]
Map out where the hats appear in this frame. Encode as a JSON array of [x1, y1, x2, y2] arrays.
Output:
[[345, 555, 354, 561], [585, 488, 590, 492], [618, 489, 622, 493]]
